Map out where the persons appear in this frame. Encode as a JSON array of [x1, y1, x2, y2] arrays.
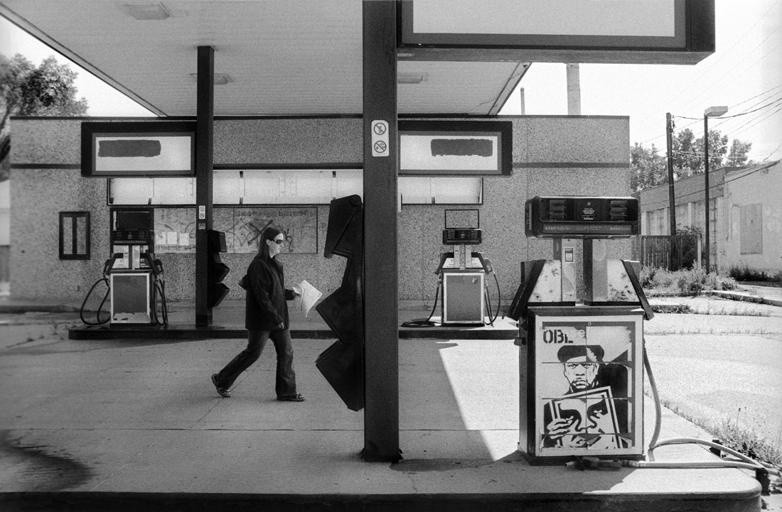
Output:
[[543, 344, 633, 447], [209, 230, 306, 402]]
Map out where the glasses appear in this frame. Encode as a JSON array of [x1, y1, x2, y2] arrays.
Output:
[[271, 239, 283, 244]]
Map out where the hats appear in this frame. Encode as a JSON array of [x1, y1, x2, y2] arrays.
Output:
[[558, 345, 604, 362]]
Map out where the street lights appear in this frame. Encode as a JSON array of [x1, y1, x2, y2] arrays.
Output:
[[703, 106, 729, 273]]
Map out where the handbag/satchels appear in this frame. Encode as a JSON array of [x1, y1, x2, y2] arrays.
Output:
[[238, 275, 246, 289]]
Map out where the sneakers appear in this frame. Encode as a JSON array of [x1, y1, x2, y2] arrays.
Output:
[[211, 374, 231, 397], [277, 393, 304, 401]]
